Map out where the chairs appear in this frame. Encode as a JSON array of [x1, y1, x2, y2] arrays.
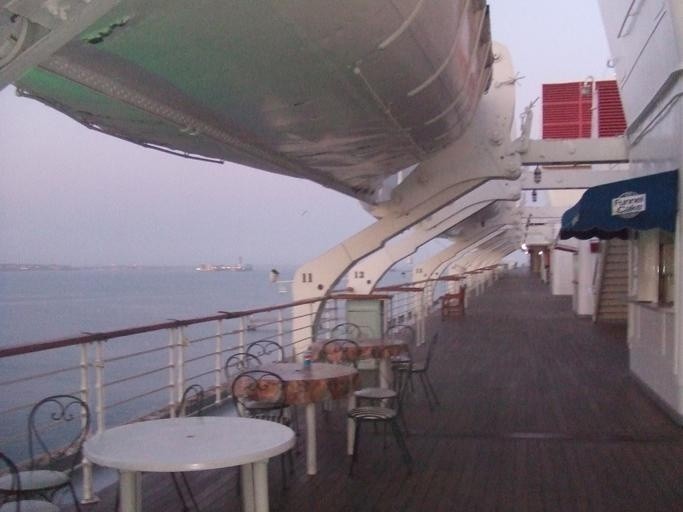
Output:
[[116, 380, 207, 510], [1, 394, 91, 512], [440, 284, 466, 321], [224, 340, 295, 494], [305, 324, 441, 474]]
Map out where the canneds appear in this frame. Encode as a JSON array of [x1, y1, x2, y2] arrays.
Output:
[[303, 351, 313, 367]]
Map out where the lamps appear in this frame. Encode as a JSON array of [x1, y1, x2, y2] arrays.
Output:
[[531, 189, 537, 202], [534, 164, 541, 184]]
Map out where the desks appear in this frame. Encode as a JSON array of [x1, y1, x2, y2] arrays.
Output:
[[224, 361, 363, 474], [75, 415, 296, 512]]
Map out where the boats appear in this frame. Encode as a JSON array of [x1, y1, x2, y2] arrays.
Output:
[[196, 264, 250, 272]]
